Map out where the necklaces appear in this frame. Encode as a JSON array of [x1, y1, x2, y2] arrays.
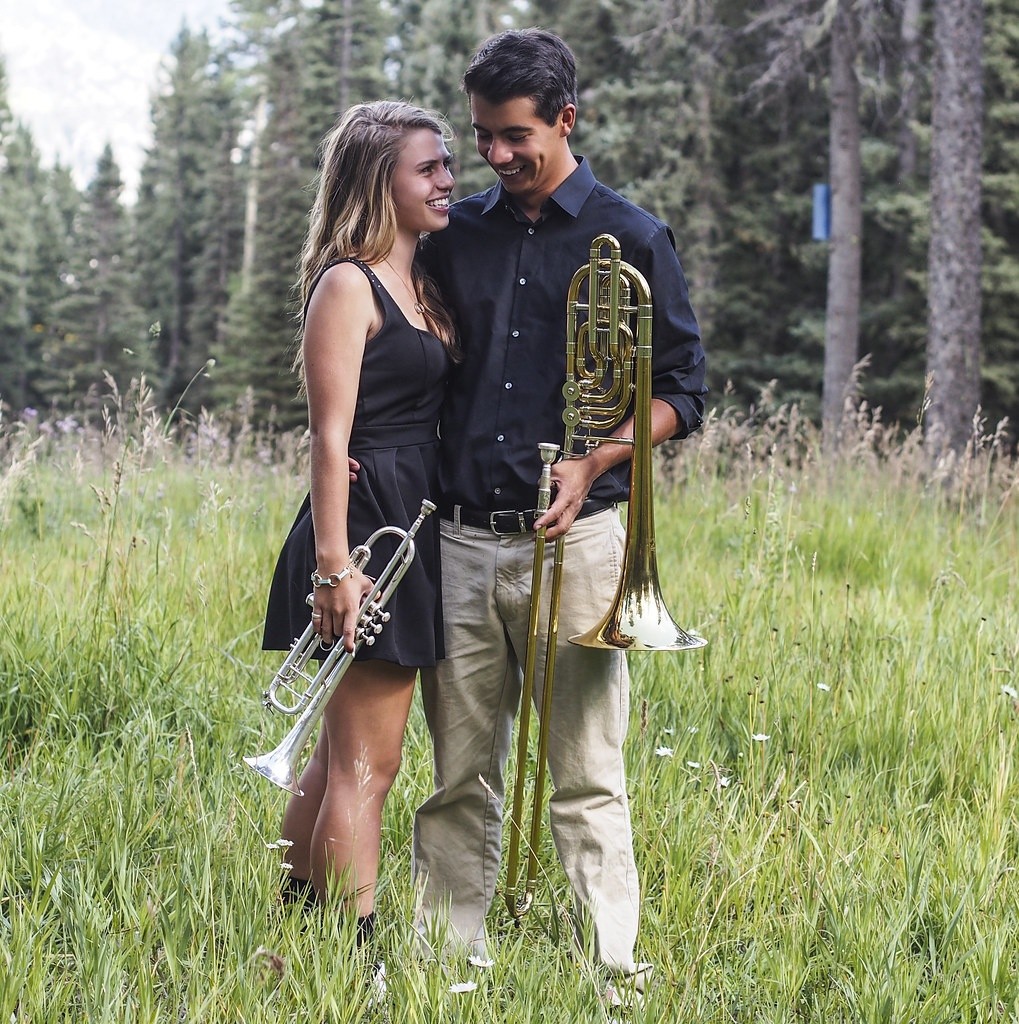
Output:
[[378, 252, 426, 314]]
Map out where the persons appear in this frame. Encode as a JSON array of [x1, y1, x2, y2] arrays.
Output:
[[259, 97, 457, 972], [342, 30, 714, 1012]]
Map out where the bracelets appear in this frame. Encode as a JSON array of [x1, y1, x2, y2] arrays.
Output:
[[310, 562, 355, 588]]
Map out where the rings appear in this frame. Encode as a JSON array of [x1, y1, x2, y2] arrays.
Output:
[[311, 612, 323, 620]]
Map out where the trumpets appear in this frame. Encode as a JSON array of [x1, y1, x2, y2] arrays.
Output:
[[242, 499, 436, 797]]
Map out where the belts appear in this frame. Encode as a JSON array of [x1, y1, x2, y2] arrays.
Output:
[[438, 494, 615, 535]]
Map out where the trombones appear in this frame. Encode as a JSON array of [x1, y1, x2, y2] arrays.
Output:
[[501, 233, 710, 921]]
